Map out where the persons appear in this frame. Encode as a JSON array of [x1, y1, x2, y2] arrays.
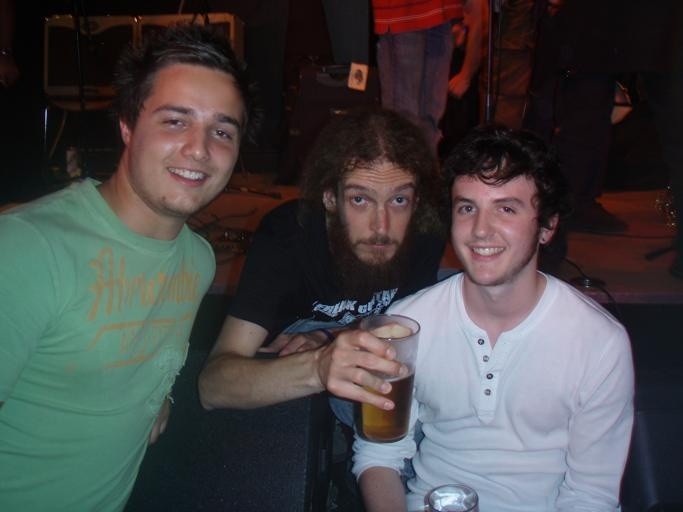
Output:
[[350, 124, 635, 512], [196, 116, 446, 512], [0, 0, 21, 87]]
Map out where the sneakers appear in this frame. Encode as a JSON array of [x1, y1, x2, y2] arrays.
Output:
[[565, 201, 628, 233]]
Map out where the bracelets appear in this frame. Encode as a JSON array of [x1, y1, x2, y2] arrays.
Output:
[[320, 328, 336, 344]]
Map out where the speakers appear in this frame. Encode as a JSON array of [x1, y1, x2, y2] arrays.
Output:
[[137, 12, 245, 100], [43, 15, 137, 98]]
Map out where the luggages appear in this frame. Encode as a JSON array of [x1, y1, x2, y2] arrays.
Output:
[[281, 62, 379, 180]]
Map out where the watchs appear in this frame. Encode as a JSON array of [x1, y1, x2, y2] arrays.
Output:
[[0, 31, 252, 512], [0, 48, 15, 56]]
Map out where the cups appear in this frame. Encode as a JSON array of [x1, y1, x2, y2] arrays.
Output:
[[423, 480, 480, 512], [358, 313, 422, 444]]
